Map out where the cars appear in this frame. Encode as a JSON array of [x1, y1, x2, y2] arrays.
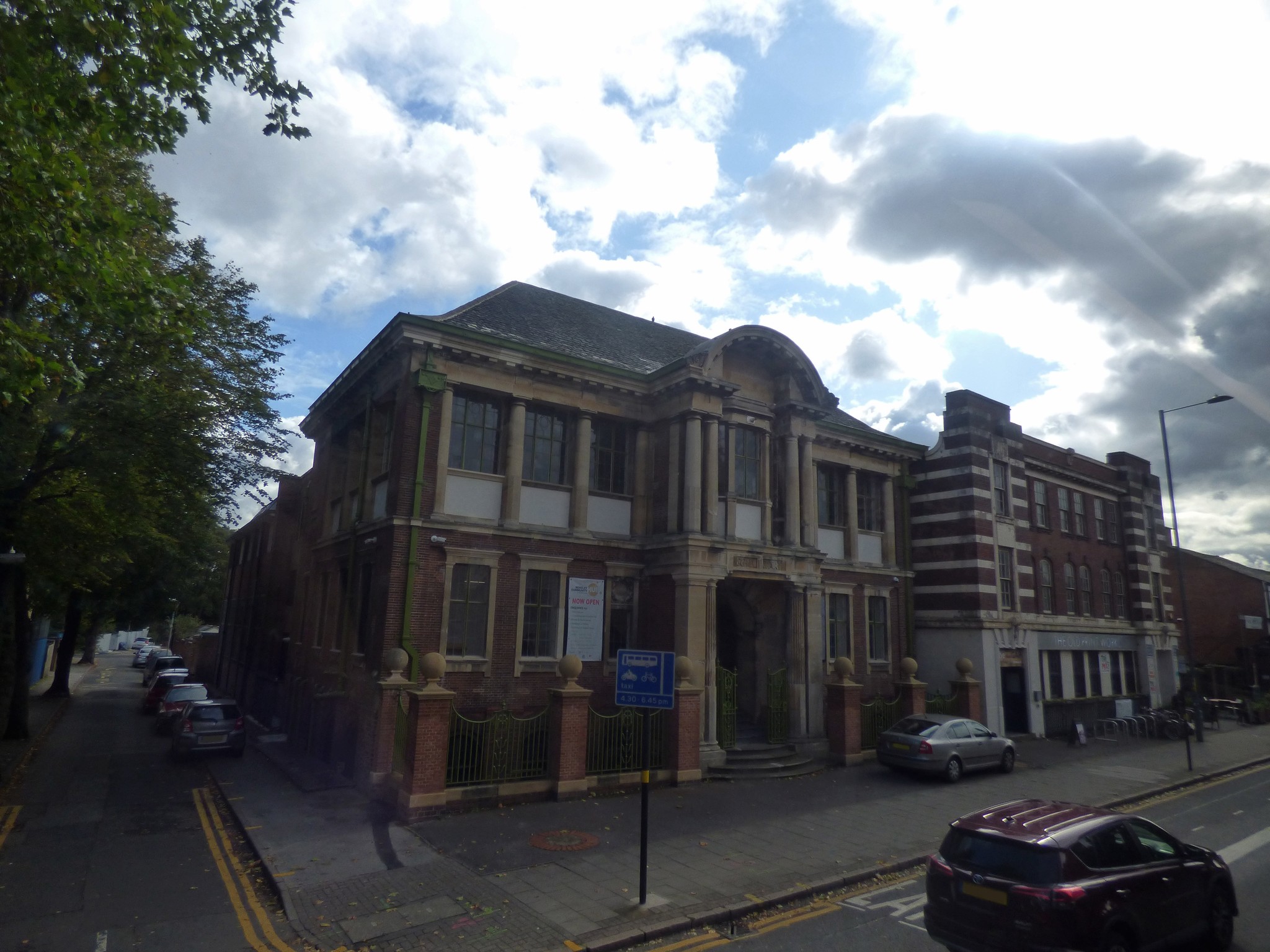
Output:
[[875, 712, 1017, 781], [142, 668, 190, 715], [133, 648, 152, 666], [132, 637, 155, 649], [143, 646, 162, 653], [145, 649, 186, 686], [174, 699, 248, 758], [159, 684, 215, 732]]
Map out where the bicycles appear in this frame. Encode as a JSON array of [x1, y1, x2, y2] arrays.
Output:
[[1137, 707, 1182, 740]]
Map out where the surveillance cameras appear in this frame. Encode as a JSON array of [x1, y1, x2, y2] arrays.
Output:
[[437, 538, 447, 542], [895, 578, 899, 583], [364, 539, 375, 544]]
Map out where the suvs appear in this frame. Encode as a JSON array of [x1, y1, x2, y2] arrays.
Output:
[[924, 797, 1238, 952]]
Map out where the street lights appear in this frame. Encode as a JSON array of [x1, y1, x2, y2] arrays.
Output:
[[1159, 394, 1231, 763], [168, 598, 180, 649]]
